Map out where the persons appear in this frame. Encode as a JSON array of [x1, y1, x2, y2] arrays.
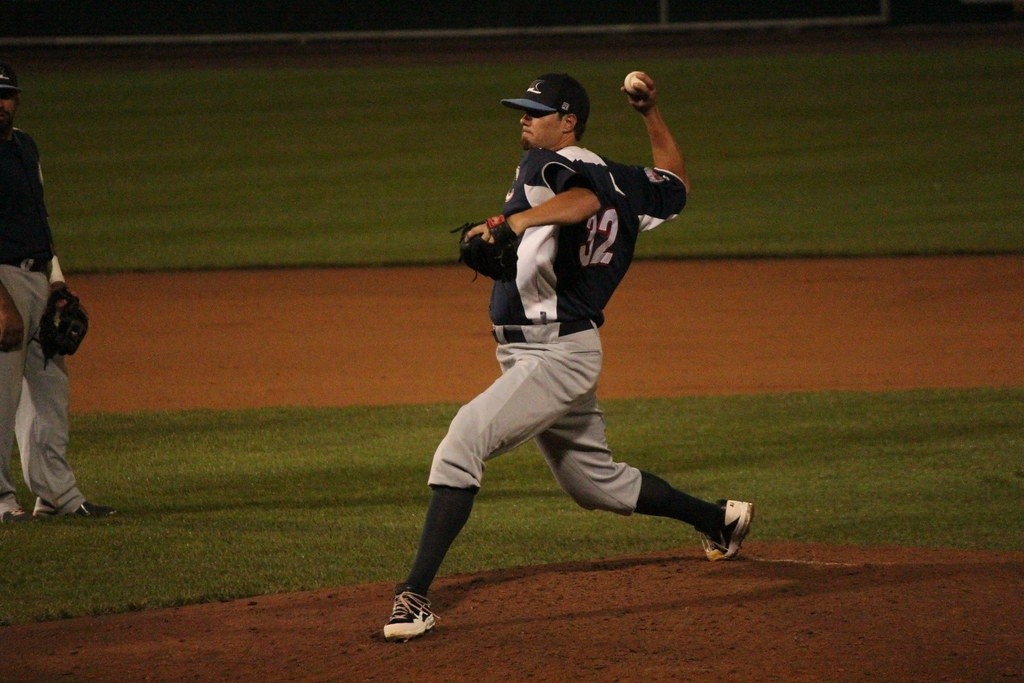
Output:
[[384, 71, 753, 638], [0, 59, 118, 522]]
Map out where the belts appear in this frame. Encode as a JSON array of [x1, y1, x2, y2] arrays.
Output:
[[490, 317, 594, 344]]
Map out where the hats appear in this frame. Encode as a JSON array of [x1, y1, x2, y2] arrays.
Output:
[[498, 73, 591, 115], [0, 62, 24, 94]]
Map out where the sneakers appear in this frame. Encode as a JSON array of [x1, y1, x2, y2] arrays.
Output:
[[383, 582, 442, 646], [32, 496, 119, 524], [1, 491, 29, 524], [699, 498, 755, 563]]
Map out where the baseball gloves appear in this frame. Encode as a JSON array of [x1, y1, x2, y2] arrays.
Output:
[[39, 285, 89, 368], [451, 219, 516, 283]]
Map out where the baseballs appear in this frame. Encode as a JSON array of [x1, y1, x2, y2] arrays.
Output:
[[623, 69, 648, 96]]
